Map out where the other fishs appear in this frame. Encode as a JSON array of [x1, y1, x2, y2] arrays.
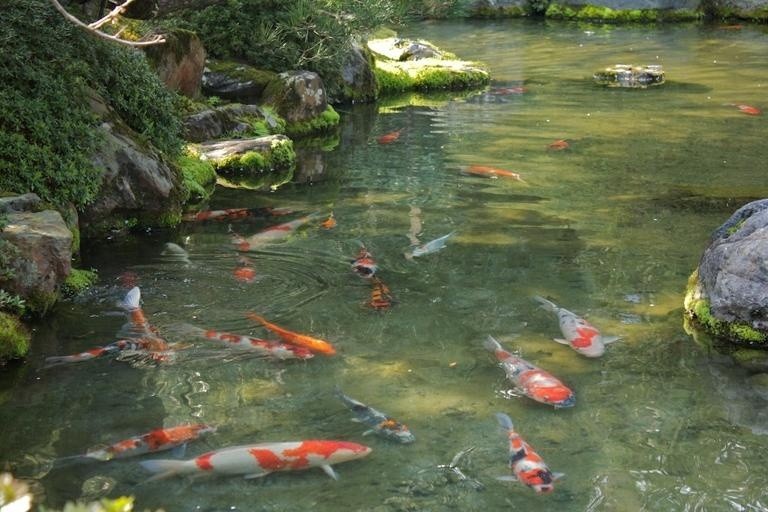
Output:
[[15, 417, 377, 484]]
